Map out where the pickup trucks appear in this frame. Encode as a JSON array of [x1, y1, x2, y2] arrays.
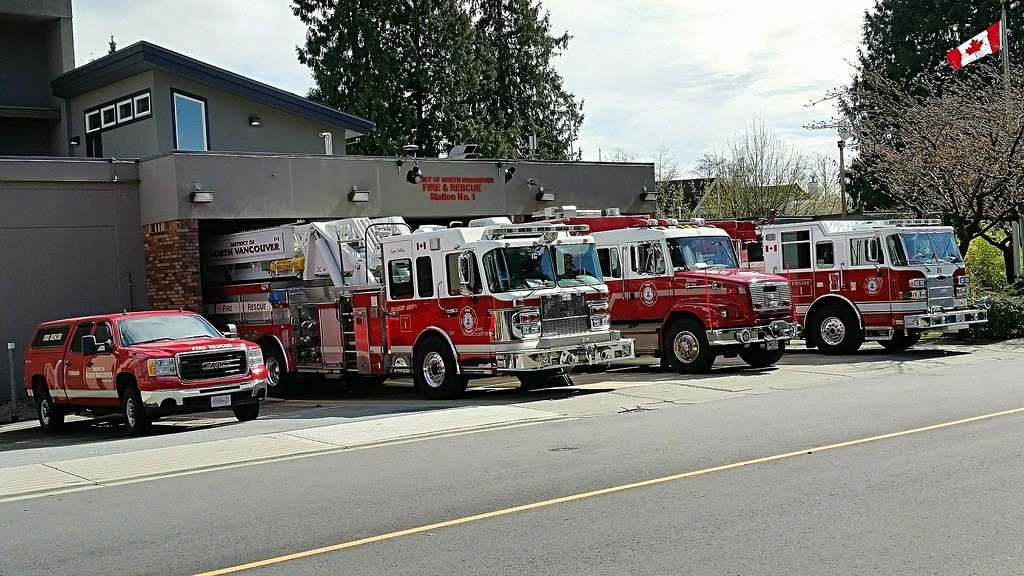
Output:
[[24, 310, 267, 436]]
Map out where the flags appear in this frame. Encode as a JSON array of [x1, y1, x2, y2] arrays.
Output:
[[944, 18, 1003, 71]]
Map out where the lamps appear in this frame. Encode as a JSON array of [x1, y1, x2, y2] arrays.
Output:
[[69, 136, 80, 146], [398, 155, 422, 184], [536, 186, 556, 201], [189, 184, 215, 203], [348, 185, 371, 202], [640, 186, 659, 201], [497, 156, 517, 184], [249, 117, 260, 126]]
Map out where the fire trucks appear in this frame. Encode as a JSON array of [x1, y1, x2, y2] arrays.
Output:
[[527, 205, 804, 376], [202, 216, 636, 400], [704, 208, 993, 357]]
